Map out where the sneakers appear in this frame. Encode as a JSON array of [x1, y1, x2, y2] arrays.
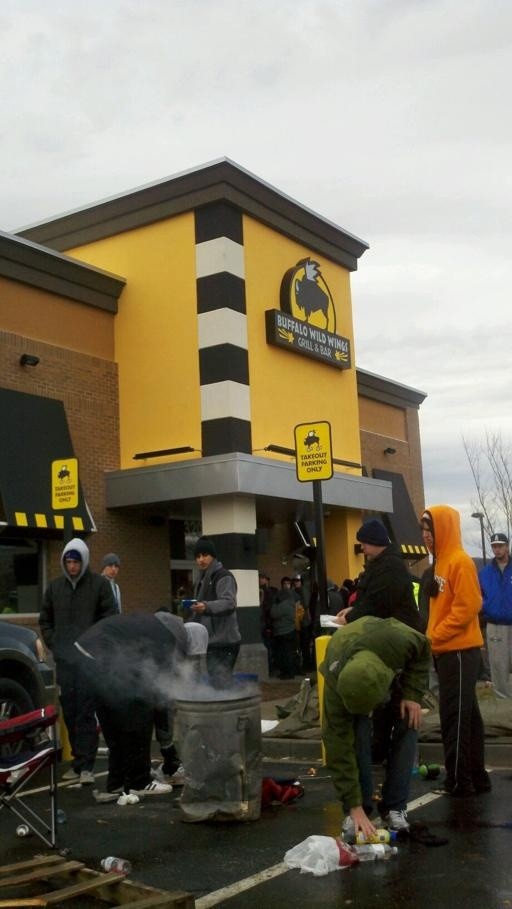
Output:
[[128, 780, 173, 796], [340, 814, 356, 843], [79, 768, 96, 784], [162, 763, 184, 786], [430, 783, 476, 796], [63, 769, 78, 779], [380, 809, 410, 831]]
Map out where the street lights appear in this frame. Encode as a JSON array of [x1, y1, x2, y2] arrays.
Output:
[[471, 511, 487, 568]]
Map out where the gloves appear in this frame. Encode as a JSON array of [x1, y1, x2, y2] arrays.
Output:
[[161, 745, 181, 773]]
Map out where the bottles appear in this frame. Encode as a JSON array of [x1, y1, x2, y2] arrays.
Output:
[[419, 761, 440, 780], [16, 824, 29, 837], [351, 830, 398, 863], [100, 856, 132, 876]]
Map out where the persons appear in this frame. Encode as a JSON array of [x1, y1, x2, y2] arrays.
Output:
[[189, 539, 241, 690], [71, 611, 210, 795], [418, 505, 491, 797], [176, 586, 193, 623], [39, 538, 118, 783], [259, 571, 360, 680], [337, 520, 420, 764], [102, 552, 121, 614], [320, 614, 433, 843], [480, 534, 512, 698]]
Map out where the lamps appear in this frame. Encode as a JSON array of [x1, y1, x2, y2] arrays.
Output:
[[18, 353, 40, 368], [384, 447, 396, 455]]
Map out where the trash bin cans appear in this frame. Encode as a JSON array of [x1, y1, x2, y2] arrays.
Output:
[[178, 692, 265, 820]]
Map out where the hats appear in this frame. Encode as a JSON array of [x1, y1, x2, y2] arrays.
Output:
[[101, 552, 120, 569], [193, 535, 217, 559], [64, 551, 81, 562], [490, 533, 508, 545], [356, 518, 389, 547], [292, 574, 302, 581]]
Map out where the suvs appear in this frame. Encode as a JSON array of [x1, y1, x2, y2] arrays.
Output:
[[0, 619, 59, 792]]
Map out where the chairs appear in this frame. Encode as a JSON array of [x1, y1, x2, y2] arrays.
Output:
[[0, 705, 64, 851]]
